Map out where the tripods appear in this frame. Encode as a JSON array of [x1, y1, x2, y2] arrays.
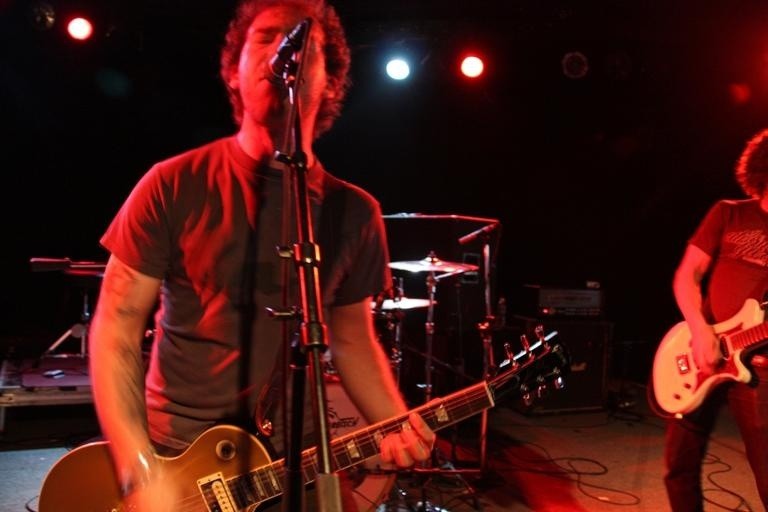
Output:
[[33, 276, 91, 368], [379, 273, 480, 512]]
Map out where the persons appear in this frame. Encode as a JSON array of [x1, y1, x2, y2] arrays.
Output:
[[655, 128, 768, 512], [78, 0, 442, 509]]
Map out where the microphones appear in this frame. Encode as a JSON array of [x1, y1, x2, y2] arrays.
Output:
[[348, 465, 367, 478], [269, 21, 307, 78]]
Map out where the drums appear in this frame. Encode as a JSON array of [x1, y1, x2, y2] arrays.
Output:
[[323, 374, 401, 511]]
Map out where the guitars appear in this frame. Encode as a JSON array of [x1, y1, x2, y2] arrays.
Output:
[[651, 299, 768, 417], [39, 324, 574, 512]]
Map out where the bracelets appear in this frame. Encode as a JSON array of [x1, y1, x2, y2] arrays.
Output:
[[117, 469, 165, 496]]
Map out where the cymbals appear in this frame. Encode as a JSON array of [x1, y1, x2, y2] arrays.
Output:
[[389, 258, 481, 280], [371, 297, 437, 310]]
